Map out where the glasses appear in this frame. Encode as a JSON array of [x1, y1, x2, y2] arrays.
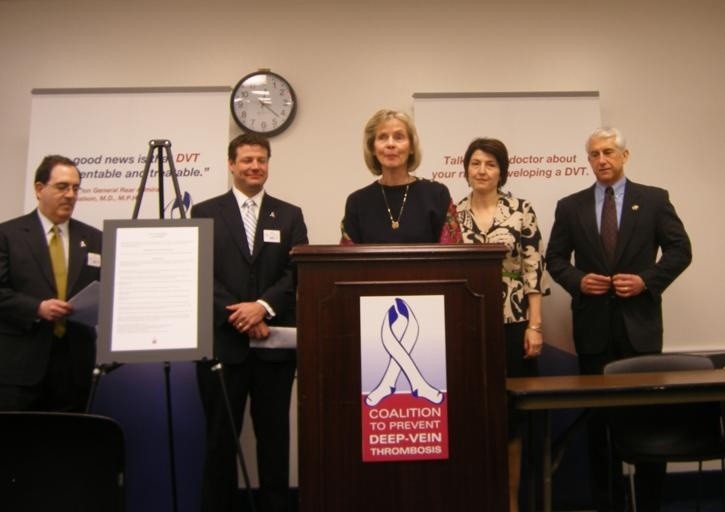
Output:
[[43, 184, 82, 193]]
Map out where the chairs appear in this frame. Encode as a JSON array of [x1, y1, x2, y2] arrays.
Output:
[[542, 342, 584, 512], [0, 410, 129, 511], [603, 353, 725, 512]]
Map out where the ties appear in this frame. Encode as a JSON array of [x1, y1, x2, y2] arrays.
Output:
[[601, 187, 619, 270], [243, 198, 258, 255], [47, 225, 68, 339]]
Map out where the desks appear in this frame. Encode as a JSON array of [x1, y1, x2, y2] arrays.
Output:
[[503, 368, 725, 512]]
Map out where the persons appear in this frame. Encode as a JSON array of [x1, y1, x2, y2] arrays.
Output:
[[0, 153, 125, 415], [191, 131, 309, 511], [454, 137, 550, 509], [544, 127, 694, 511], [337, 107, 463, 246]]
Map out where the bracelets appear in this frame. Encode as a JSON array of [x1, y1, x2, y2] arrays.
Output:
[[527, 324, 544, 333]]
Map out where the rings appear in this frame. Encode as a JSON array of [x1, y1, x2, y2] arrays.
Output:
[[239, 321, 243, 327], [624, 286, 628, 292]]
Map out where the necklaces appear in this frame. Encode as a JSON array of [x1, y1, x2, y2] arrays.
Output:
[[379, 173, 410, 231]]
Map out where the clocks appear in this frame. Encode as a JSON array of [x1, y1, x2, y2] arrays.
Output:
[[229, 69, 298, 137]]
